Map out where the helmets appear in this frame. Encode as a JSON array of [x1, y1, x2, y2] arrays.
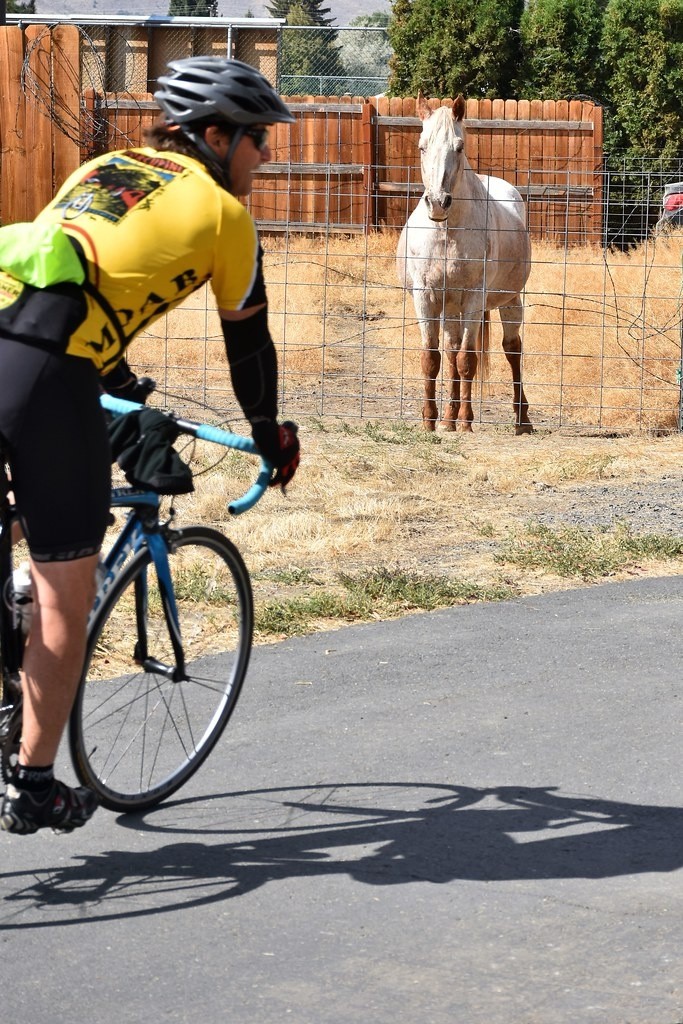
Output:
[[155, 56, 298, 125]]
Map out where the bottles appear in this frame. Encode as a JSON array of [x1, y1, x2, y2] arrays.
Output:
[[12, 564, 32, 632], [95, 552, 108, 592]]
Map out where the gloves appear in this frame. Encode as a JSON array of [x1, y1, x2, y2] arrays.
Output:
[[254, 418, 301, 486]]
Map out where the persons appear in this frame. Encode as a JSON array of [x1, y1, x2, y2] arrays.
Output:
[[0, 55, 302, 835]]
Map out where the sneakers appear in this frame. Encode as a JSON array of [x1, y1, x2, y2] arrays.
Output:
[[0, 776, 102, 834]]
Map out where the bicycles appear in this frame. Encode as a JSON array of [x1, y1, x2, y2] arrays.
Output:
[[0, 392, 289, 835]]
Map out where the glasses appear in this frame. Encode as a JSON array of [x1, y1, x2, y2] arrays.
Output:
[[224, 122, 267, 151]]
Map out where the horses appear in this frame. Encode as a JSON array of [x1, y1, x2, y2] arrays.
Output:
[[392, 87, 536, 437]]
